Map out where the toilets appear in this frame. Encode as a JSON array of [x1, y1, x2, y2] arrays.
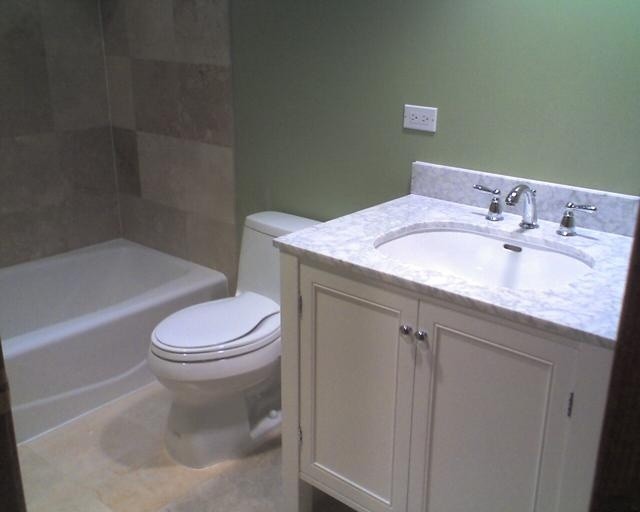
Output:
[[149, 212, 325, 471]]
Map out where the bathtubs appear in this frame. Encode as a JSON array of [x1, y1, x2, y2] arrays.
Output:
[[1, 238, 227, 448]]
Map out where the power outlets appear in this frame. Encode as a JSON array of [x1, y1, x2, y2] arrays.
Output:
[[403, 104, 437, 133]]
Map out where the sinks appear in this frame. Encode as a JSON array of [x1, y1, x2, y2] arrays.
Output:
[[371, 217, 599, 296]]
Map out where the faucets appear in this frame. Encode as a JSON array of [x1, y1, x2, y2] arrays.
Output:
[[505, 184, 539, 229]]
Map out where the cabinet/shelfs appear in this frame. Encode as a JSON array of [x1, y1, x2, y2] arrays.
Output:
[[298, 263, 581, 512]]
